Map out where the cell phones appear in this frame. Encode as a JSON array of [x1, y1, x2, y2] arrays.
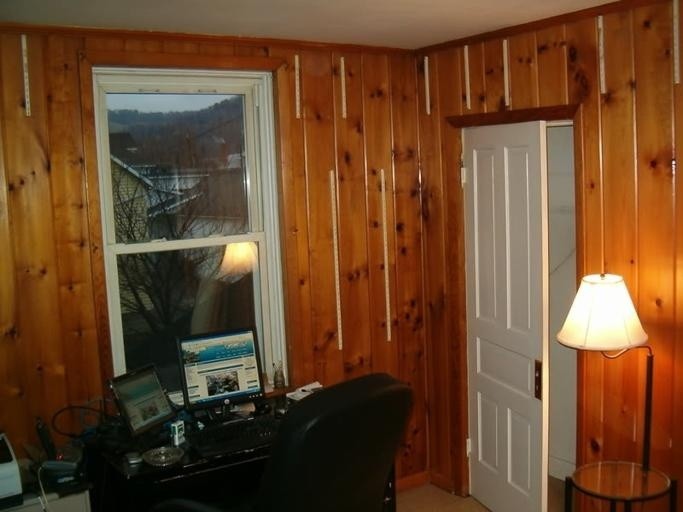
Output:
[[125, 450, 142, 463]]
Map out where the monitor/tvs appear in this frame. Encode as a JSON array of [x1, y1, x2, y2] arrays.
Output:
[[176, 327, 266, 409], [109, 363, 176, 437]]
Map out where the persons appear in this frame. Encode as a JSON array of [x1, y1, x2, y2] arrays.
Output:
[[208, 376, 238, 396]]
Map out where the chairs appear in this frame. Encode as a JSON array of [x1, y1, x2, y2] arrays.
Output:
[[157, 373, 412, 511]]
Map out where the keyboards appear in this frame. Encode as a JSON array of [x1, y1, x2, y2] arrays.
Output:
[[190, 414, 281, 459]]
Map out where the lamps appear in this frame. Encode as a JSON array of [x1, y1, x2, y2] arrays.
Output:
[[214, 240, 261, 274], [556, 272, 655, 471]]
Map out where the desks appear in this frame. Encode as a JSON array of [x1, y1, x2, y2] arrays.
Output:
[[565, 461, 678, 512], [82, 395, 303, 511]]
